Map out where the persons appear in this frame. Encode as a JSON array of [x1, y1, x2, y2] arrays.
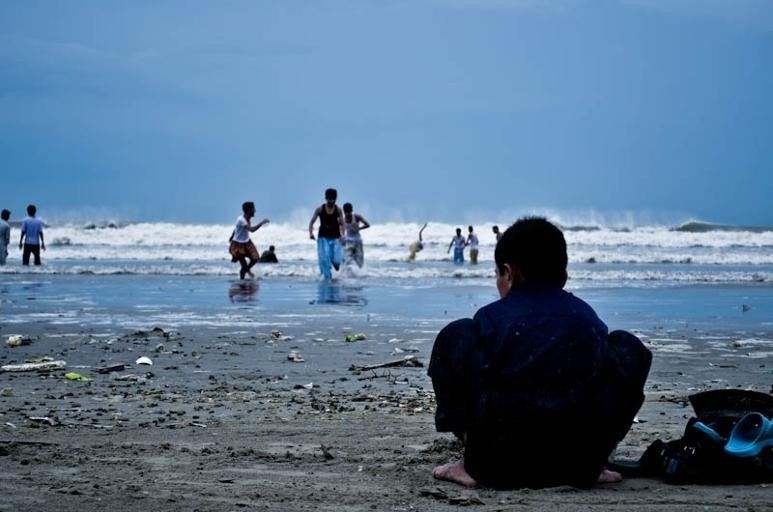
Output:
[[0, 208, 12, 265], [343, 202, 370, 269], [309, 188, 346, 282], [259, 245, 278, 263], [447, 225, 503, 265], [19, 204, 46, 266], [426, 215, 654, 490], [228, 201, 270, 281]]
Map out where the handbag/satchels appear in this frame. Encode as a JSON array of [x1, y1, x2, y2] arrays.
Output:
[[611, 388, 772, 486]]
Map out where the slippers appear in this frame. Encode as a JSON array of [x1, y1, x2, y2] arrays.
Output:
[[723, 411, 772, 459], [689, 422, 723, 444]]
